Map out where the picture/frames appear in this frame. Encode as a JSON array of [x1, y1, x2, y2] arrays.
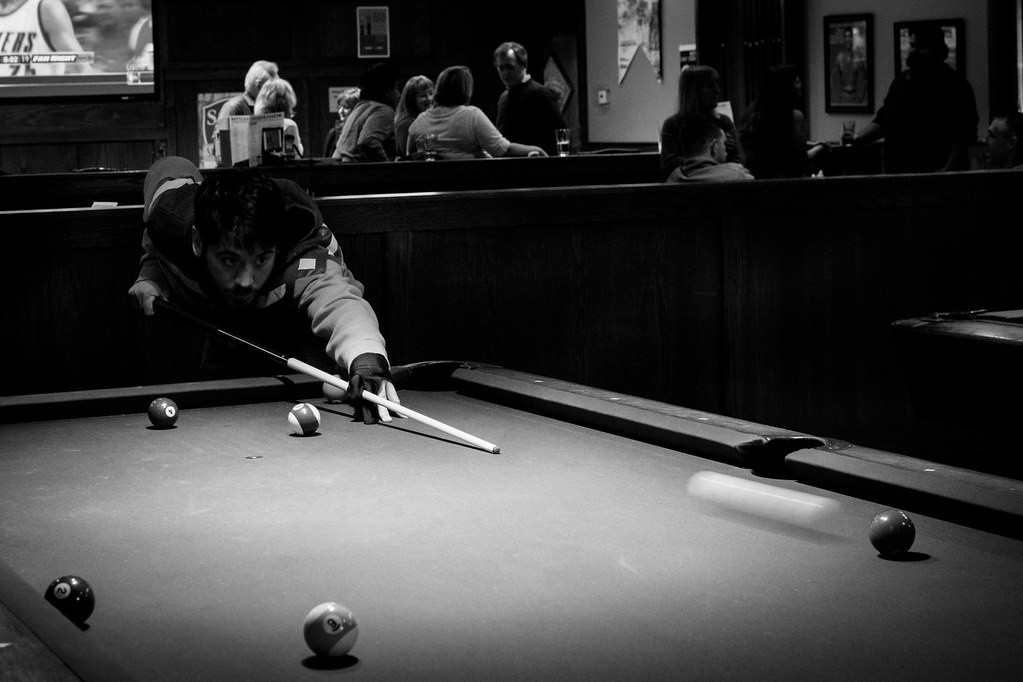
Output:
[[356, 6, 389, 58], [893, 18, 966, 76], [823, 12, 874, 115]]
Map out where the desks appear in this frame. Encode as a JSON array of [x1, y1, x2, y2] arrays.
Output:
[[0, 311, 1023, 682]]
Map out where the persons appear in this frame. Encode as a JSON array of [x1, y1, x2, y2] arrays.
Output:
[[661, 24, 1023, 184], [128, 156, 410, 426], [836, 27, 860, 103], [211, 59, 306, 165], [324, 41, 581, 161], [0, 0, 153, 77]]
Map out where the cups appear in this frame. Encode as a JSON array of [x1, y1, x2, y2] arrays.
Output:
[[842, 120, 856, 138], [423, 132, 438, 162], [555, 128, 571, 157]]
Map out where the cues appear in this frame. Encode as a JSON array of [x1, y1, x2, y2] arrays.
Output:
[[151, 297, 501, 455]]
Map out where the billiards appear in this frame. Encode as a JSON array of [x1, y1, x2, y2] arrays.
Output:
[[289, 403, 321, 436], [303, 602, 359, 658], [44, 576, 96, 625], [869, 511, 916, 554], [323, 374, 349, 403], [148, 398, 179, 428]]
